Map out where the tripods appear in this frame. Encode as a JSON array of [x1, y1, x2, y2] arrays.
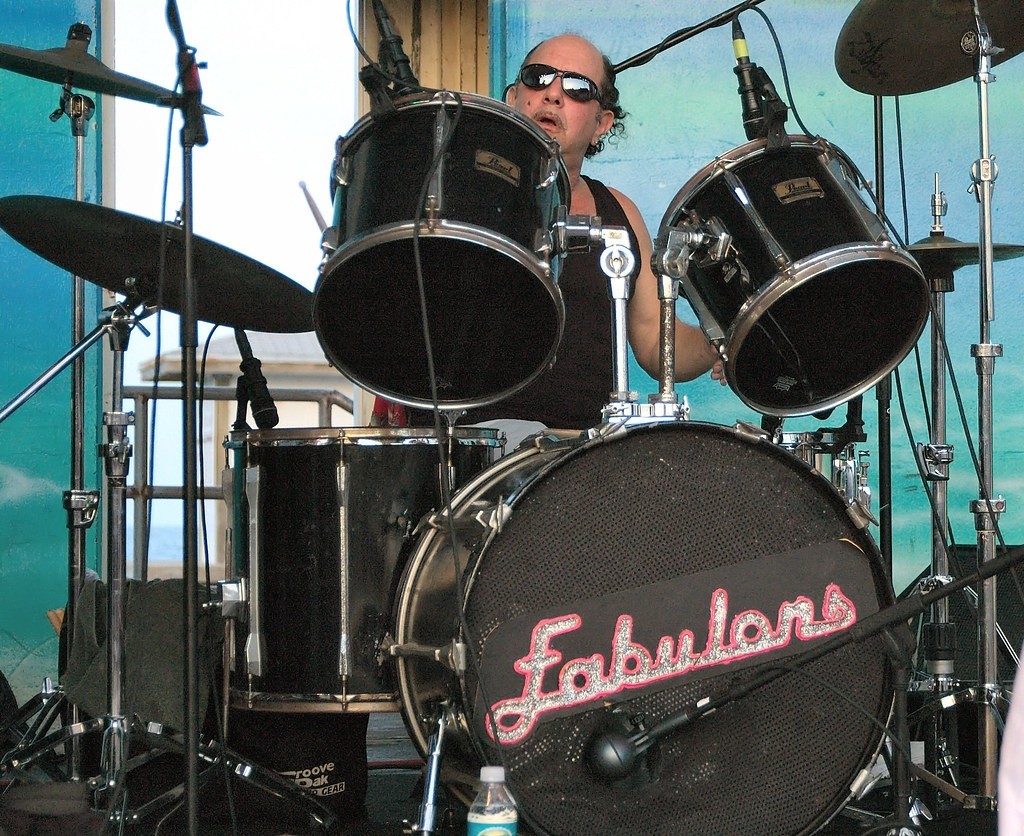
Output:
[[893, 11, 1023, 836], [0, 78, 346, 836]]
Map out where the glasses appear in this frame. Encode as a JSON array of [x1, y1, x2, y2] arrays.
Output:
[[515, 63, 603, 104]]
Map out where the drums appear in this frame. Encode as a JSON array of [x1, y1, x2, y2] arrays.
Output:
[[390, 420, 903, 836], [309, 88, 573, 411], [655, 133, 931, 420], [222, 425, 500, 717]]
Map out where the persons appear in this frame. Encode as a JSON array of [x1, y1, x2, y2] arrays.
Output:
[[406, 35, 727, 429]]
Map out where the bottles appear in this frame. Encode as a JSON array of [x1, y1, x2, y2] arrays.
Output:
[[468, 766, 517, 836]]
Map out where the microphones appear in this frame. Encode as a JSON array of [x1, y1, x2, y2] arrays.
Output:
[[587, 697, 716, 783], [731, 18, 765, 141], [167, 0, 208, 147], [371, 0, 419, 90], [233, 328, 280, 429]]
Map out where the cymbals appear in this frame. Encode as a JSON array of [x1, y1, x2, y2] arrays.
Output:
[[0, 39, 226, 118], [902, 235, 1024, 269], [834, 0, 1024, 98], [0, 193, 319, 334]]
